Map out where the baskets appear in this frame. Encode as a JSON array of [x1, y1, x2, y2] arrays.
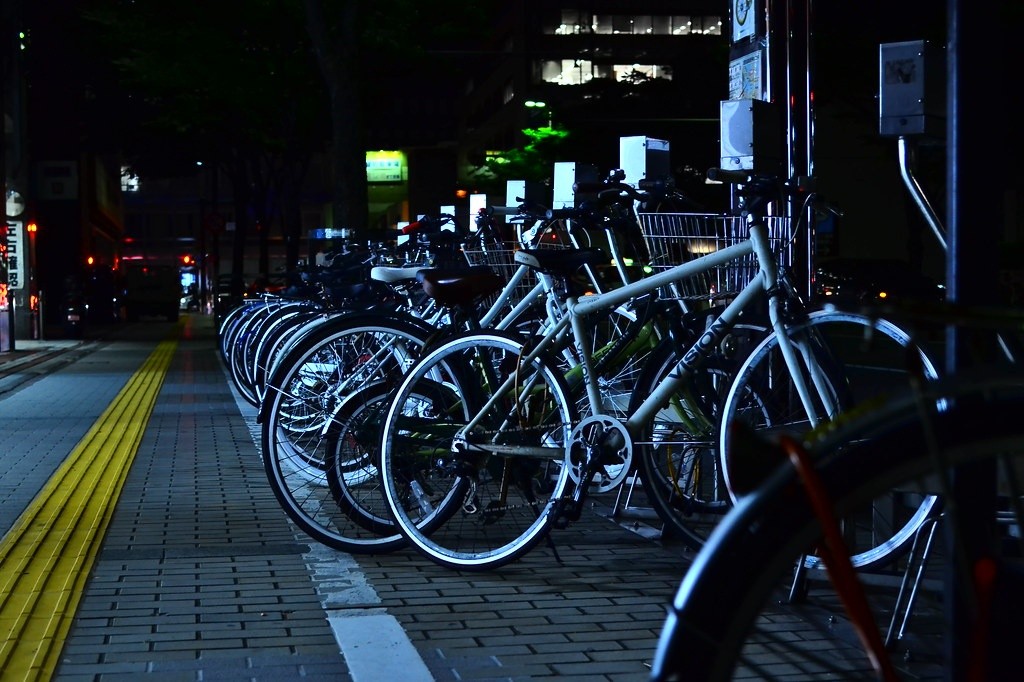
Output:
[[460, 246, 565, 311], [642, 210, 792, 297]]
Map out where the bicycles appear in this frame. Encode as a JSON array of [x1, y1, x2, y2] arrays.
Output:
[[218, 170, 1024, 682]]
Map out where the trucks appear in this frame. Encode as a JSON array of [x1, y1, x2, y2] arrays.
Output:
[[128, 271, 183, 321]]
